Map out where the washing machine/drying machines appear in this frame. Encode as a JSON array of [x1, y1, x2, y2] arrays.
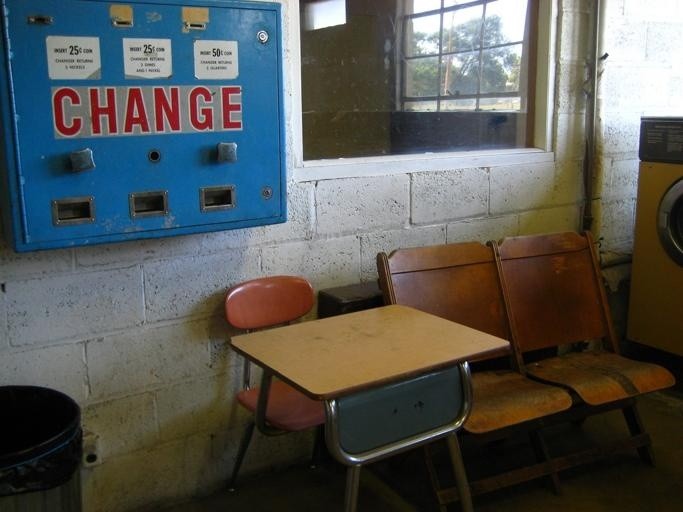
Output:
[[625, 115, 683, 358]]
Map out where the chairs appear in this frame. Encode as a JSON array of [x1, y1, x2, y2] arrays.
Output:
[[222, 275, 326, 499], [374, 240, 573, 512], [485, 228, 675, 500]]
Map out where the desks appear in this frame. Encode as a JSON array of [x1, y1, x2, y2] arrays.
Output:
[[228, 302, 513, 511]]
